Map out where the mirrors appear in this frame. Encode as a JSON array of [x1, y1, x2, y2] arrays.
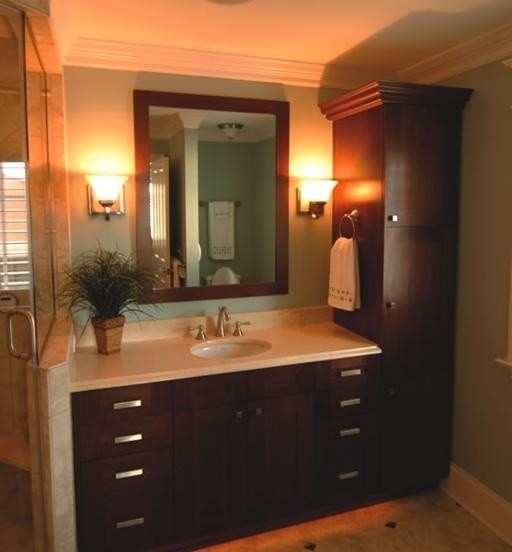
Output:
[[131, 87, 291, 304]]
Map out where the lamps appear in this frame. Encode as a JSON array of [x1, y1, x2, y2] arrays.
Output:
[[296, 179, 340, 220], [217, 123, 244, 140], [84, 174, 128, 222]]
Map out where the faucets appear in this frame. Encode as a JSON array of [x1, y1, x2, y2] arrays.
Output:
[[216, 306, 231, 336]]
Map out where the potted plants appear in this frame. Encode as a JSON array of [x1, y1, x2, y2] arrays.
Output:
[[54, 239, 169, 355]]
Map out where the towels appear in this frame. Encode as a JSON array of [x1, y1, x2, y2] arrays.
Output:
[[325, 237, 361, 312], [170, 254, 186, 289], [207, 201, 235, 260]]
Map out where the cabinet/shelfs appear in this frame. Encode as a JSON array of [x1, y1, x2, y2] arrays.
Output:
[[71, 356, 384, 552], [318, 80, 475, 504]]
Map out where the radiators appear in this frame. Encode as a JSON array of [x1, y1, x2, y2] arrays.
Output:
[[148, 152, 171, 269]]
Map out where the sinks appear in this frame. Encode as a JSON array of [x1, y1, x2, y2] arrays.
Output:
[[188, 334, 274, 362]]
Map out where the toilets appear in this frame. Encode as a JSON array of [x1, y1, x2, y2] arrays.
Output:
[[202, 267, 242, 287]]
